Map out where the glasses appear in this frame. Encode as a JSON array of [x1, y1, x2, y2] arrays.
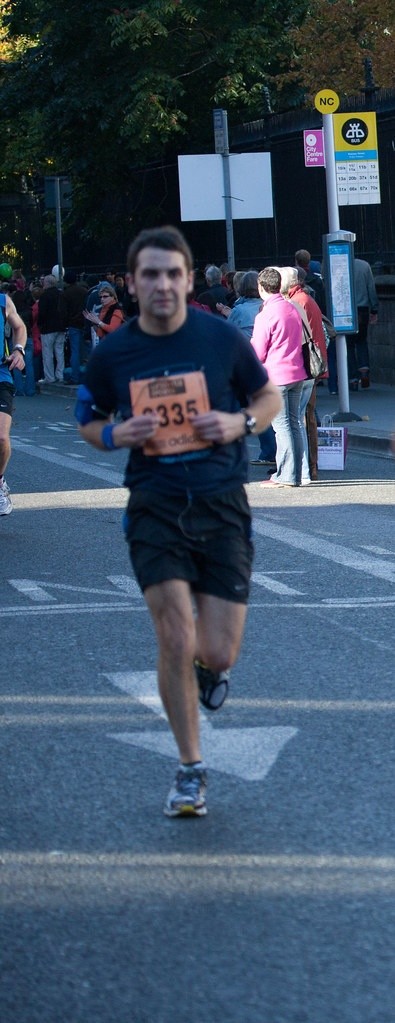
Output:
[[100, 295, 109, 298]]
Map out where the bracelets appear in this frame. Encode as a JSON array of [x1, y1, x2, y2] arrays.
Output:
[[13, 344, 26, 355], [101, 421, 117, 452]]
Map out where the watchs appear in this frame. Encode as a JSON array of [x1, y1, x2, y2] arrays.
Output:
[[241, 408, 257, 436]]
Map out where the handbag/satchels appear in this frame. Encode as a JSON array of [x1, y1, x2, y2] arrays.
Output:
[[288, 300, 325, 378]]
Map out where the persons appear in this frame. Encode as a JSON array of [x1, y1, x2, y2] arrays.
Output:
[[248, 269, 311, 485], [188, 243, 379, 485], [5, 261, 140, 399], [0, 293, 28, 516], [70, 228, 282, 815]]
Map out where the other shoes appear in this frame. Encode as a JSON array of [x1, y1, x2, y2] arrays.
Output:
[[260, 479, 292, 488], [63, 379, 81, 385], [361, 370, 369, 388], [311, 475, 317, 480], [0, 475, 12, 515], [250, 459, 276, 465], [39, 379, 55, 383], [295, 479, 310, 487], [351, 382, 359, 391]]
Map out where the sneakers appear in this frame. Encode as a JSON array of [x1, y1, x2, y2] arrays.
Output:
[[193, 654, 229, 710], [163, 762, 207, 818]]
